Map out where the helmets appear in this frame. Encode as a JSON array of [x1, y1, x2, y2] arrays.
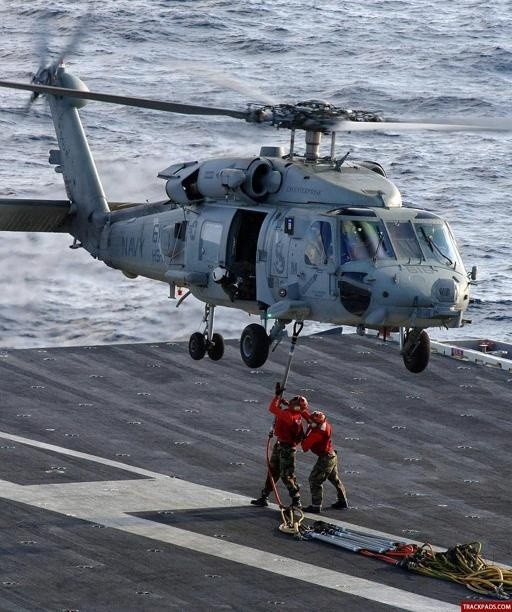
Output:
[[310, 411, 326, 427], [288, 395, 308, 413]]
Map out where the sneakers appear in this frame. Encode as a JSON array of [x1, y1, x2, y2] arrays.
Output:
[[331, 500, 348, 510], [301, 504, 322, 514], [286, 496, 303, 508], [250, 497, 268, 507]]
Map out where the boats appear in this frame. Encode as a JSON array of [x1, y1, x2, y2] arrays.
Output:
[[1, 320, 512, 612]]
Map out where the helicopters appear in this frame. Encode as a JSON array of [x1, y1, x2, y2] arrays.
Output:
[[1, 1, 511, 373]]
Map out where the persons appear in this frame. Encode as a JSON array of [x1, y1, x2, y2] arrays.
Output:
[[252, 380, 309, 512], [304, 410, 350, 514]]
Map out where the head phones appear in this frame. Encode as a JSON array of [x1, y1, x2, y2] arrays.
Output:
[[294, 396, 303, 411], [311, 415, 325, 429]]
